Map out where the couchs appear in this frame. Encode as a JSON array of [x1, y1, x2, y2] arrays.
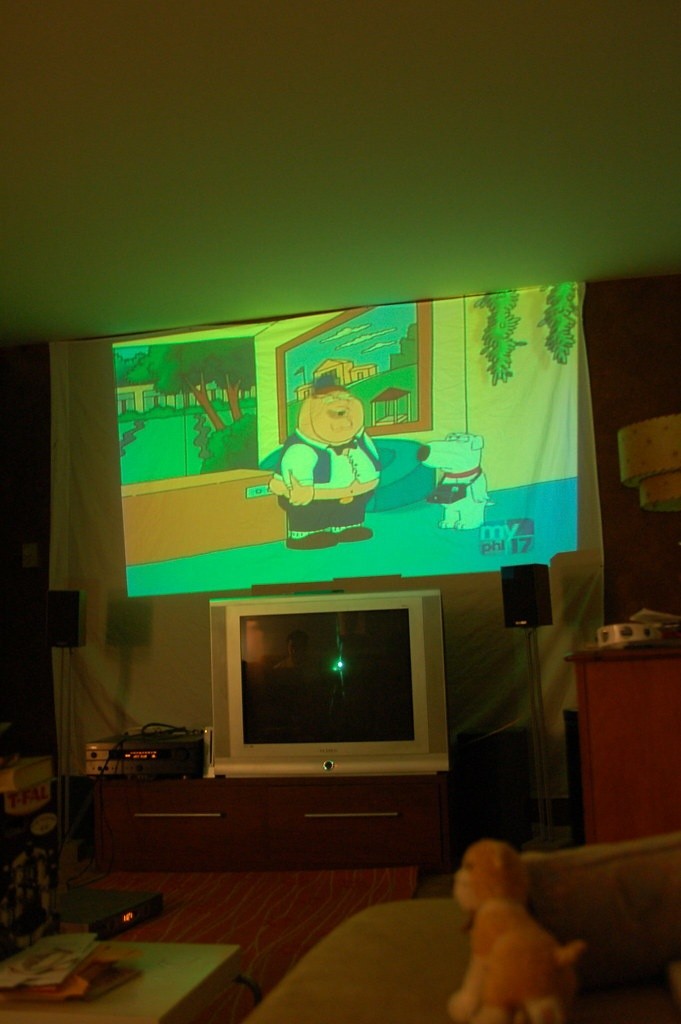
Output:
[[237, 899, 681, 1024]]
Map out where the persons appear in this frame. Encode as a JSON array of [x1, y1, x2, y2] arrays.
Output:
[[273, 630, 315, 673]]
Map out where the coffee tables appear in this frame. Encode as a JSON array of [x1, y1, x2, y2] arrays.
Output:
[[0, 942, 242, 1023]]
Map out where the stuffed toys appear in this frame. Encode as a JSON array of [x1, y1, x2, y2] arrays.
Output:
[[447, 838, 588, 1024]]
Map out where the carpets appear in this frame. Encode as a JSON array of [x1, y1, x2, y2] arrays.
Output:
[[92, 865, 420, 1024]]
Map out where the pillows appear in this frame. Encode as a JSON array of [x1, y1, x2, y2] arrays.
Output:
[[522, 831, 681, 978]]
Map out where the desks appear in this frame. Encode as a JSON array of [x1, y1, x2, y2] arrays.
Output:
[[562, 646, 681, 848]]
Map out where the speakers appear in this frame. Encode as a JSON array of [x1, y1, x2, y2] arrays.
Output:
[[47, 590, 80, 646], [501, 564, 553, 628]]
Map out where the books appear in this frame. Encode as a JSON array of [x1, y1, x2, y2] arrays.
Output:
[[1, 755, 57, 793], [0, 932, 142, 1002]]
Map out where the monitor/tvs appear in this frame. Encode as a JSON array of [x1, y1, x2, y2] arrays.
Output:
[[209, 587, 451, 777]]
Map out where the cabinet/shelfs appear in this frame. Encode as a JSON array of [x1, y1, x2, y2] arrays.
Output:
[[95, 771, 465, 876]]
